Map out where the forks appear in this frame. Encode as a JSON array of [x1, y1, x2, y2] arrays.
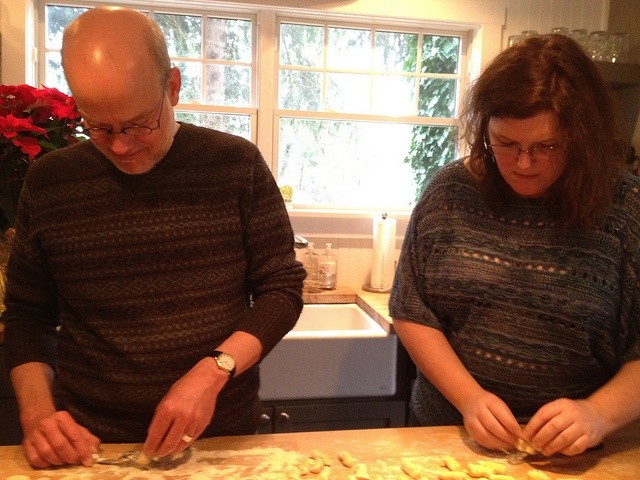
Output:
[[96, 450, 160, 470]]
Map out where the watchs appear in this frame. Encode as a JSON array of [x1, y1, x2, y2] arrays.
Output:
[[207, 351, 236, 383]]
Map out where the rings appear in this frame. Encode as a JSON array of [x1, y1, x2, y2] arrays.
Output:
[[182, 434, 192, 442]]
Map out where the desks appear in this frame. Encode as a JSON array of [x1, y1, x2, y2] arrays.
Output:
[[0, 423, 640, 480]]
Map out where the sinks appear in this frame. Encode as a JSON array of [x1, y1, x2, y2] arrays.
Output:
[[251, 304, 396, 400]]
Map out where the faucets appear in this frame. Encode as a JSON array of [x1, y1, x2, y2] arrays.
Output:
[[294, 235, 308, 248]]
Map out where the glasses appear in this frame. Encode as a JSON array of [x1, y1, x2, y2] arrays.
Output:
[[82, 84, 166, 139], [482, 131, 567, 157]]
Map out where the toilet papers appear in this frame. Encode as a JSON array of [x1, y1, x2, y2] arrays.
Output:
[[371, 218, 396, 290]]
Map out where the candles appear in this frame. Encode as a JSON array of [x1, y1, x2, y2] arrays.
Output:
[[370, 212, 397, 290]]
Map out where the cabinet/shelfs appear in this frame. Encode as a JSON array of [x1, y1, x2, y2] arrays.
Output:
[[253, 396, 407, 434]]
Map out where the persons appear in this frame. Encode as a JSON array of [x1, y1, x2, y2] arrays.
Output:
[[388, 35, 640, 457], [5, 5, 308, 468]]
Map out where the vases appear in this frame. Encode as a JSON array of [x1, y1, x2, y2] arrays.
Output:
[[0, 226, 16, 320]]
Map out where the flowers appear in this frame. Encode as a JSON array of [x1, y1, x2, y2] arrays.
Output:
[[0, 81, 84, 227]]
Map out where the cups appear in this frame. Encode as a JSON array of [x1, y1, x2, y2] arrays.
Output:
[[508, 27, 630, 63]]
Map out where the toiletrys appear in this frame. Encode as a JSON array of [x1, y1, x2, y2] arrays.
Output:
[[302, 242, 319, 291], [319, 243, 337, 289]]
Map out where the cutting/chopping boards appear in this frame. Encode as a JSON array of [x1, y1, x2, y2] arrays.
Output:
[[1, 420, 640, 479]]
